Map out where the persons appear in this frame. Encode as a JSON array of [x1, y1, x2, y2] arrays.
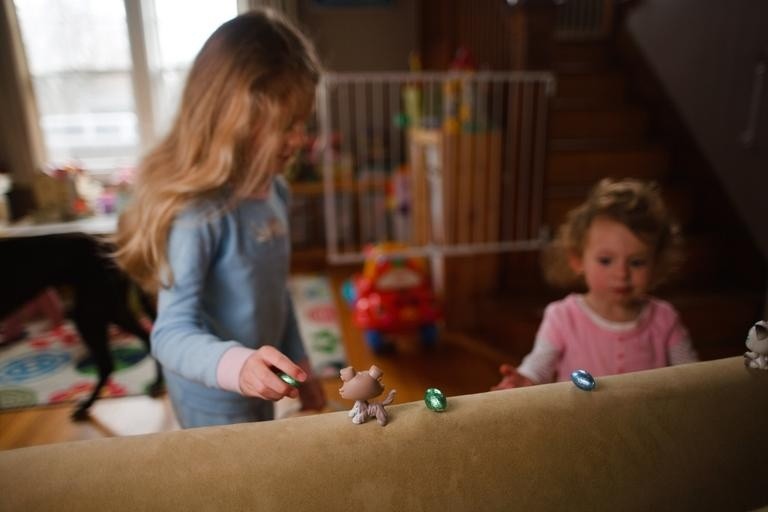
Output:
[[495, 177, 696, 391], [97, 17, 327, 431]]
